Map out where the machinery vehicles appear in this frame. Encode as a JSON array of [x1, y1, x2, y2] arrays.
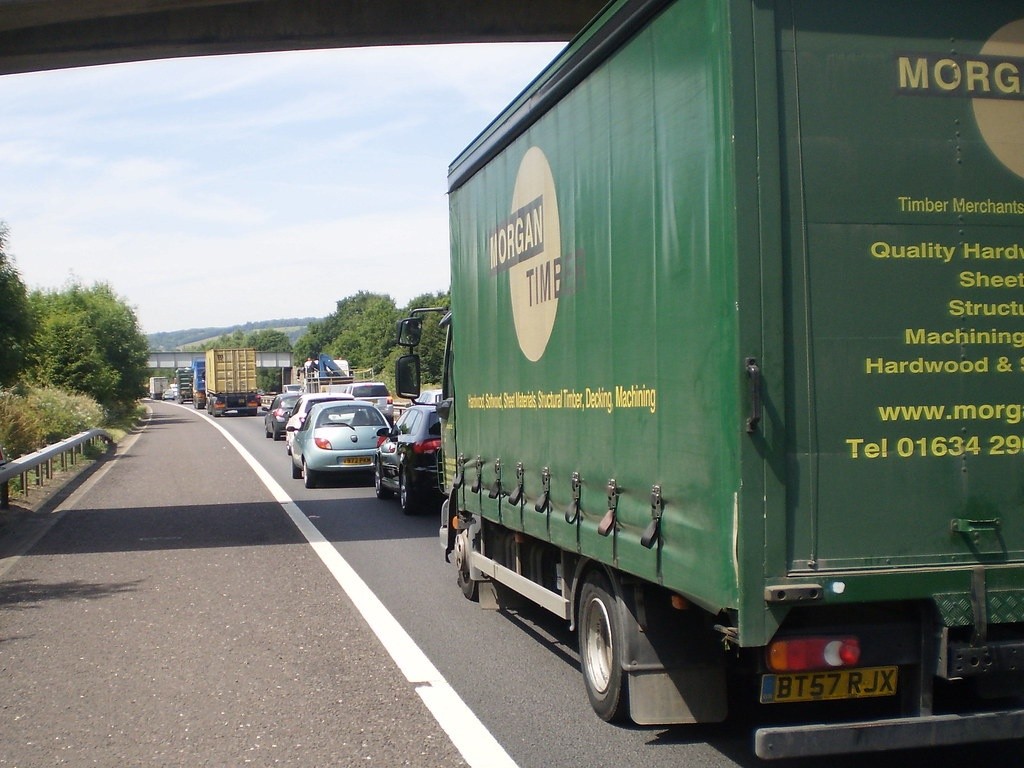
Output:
[[296, 352, 354, 393]]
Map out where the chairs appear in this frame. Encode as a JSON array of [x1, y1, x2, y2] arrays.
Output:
[[350, 411, 371, 426], [320, 413, 333, 423]]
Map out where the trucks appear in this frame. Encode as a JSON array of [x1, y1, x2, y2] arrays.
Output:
[[176, 348, 262, 417], [396, 0, 1024, 759], [170, 384, 178, 396], [149, 377, 168, 400]]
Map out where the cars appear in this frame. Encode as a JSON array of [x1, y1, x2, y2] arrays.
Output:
[[286, 393, 357, 456], [344, 382, 394, 427], [374, 389, 443, 517], [262, 393, 303, 441], [162, 389, 174, 401], [287, 401, 391, 488]]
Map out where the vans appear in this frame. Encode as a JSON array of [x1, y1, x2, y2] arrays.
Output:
[[283, 384, 303, 394]]
